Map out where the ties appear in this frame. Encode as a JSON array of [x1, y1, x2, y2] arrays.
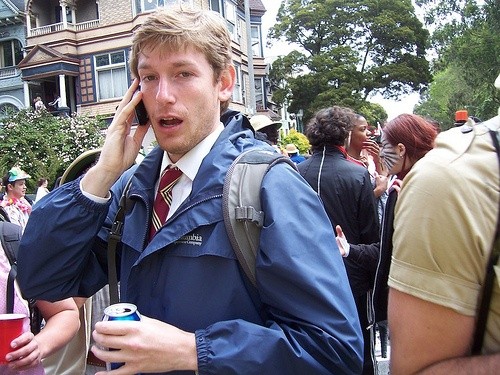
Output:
[[147, 165, 183, 243]]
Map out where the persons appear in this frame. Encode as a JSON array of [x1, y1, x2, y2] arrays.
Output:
[[249, 105, 440, 375], [15, 3, 364, 375], [388, 121, 500, 375], [48, 94, 60, 107], [34, 97, 47, 110], [0, 148, 120, 375]]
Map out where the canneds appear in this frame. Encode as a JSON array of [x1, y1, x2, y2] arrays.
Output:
[[101, 302, 143, 371]]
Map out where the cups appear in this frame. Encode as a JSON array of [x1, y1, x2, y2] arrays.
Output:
[[0, 314, 27, 365]]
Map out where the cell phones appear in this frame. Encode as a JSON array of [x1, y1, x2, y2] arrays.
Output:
[[131, 84, 149, 128]]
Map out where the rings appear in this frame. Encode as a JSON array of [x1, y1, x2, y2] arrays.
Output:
[[115, 104, 118, 110]]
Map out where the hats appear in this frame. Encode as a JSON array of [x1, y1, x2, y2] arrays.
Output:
[[7, 168, 31, 182], [249, 115, 282, 130]]
